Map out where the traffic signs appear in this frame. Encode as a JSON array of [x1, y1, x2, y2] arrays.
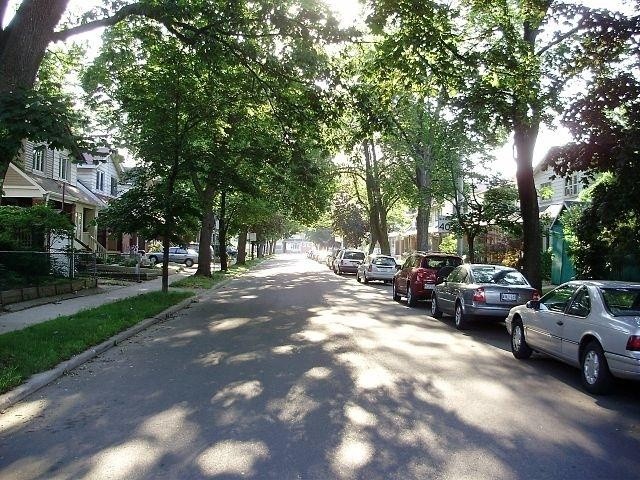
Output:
[[438, 215, 453, 232]]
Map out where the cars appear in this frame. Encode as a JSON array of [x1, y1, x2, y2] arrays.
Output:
[[306, 248, 399, 284], [431, 264, 539, 330], [145, 243, 238, 268], [392, 251, 464, 307], [505, 280, 640, 392]]
